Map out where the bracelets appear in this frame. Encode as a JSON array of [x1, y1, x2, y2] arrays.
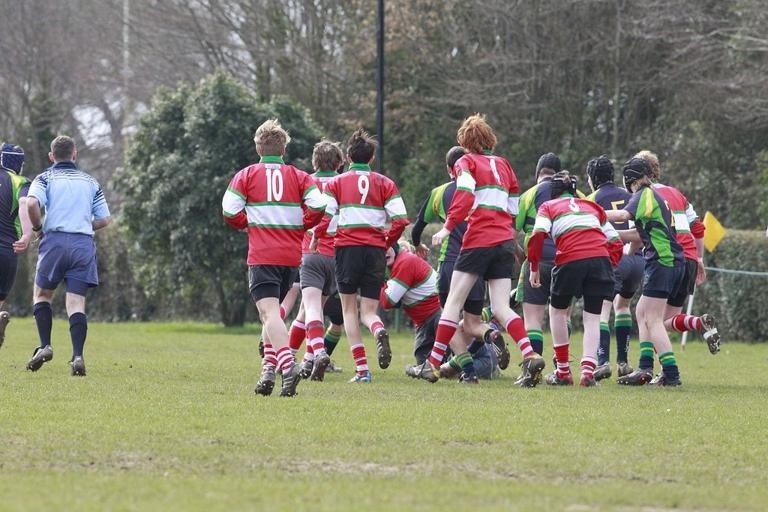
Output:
[[32, 223, 44, 231]]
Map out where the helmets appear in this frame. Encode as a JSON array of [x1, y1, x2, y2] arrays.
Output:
[[0, 142, 25, 176], [535, 152, 560, 182], [551, 170, 577, 198], [587, 154, 614, 187], [622, 158, 656, 193]]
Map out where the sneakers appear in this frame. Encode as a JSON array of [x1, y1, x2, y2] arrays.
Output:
[[697, 313, 720, 354], [347, 369, 372, 385], [592, 360, 611, 380], [406, 327, 511, 384], [514, 352, 573, 385], [580, 372, 597, 386], [68, 355, 86, 376], [616, 366, 653, 385], [374, 327, 391, 369], [0, 309, 10, 347], [255, 334, 342, 396], [644, 372, 682, 388], [25, 344, 53, 371], [616, 362, 633, 376]]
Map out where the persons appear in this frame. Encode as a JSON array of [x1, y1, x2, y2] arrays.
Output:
[[622, 150, 720, 381], [255, 112, 642, 392], [26, 135, 111, 376], [222, 118, 326, 398], [603, 158, 683, 387], [0, 141, 33, 354]]
